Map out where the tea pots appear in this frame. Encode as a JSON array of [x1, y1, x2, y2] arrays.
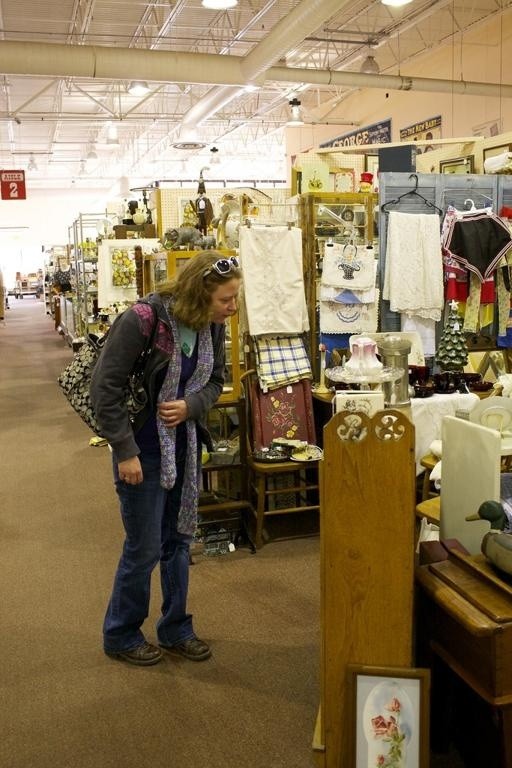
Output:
[[344, 332, 384, 377]]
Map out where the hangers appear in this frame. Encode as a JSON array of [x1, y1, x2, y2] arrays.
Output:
[[379, 171, 444, 219]]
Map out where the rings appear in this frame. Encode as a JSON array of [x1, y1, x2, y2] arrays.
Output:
[[123, 477, 130, 481]]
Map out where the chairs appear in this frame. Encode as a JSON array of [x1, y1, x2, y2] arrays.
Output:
[[241, 365, 322, 564]]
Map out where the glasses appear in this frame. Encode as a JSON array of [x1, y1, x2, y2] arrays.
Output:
[[204, 256, 239, 276]]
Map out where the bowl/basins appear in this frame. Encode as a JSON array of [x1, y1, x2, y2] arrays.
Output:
[[252, 445, 291, 462], [407, 362, 494, 398]]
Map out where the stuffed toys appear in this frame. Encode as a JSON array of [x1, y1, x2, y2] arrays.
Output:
[[193, 235, 216, 251], [164, 222, 202, 251]]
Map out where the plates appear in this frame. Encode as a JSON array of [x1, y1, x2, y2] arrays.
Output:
[[290, 454, 323, 463], [322, 364, 405, 387]]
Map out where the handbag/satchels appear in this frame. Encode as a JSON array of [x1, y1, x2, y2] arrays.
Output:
[[57, 300, 160, 438]]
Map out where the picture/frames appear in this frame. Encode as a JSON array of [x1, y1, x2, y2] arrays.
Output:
[[342, 661, 431, 768]]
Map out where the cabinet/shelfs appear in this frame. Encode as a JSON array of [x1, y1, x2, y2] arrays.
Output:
[[414, 559, 512, 768], [190, 396, 248, 564]]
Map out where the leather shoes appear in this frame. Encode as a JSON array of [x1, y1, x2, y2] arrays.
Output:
[[174, 635, 211, 660], [105, 641, 163, 665]]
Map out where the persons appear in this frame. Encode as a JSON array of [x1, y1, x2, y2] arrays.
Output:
[[87, 249, 241, 668], [52, 259, 76, 294]]
